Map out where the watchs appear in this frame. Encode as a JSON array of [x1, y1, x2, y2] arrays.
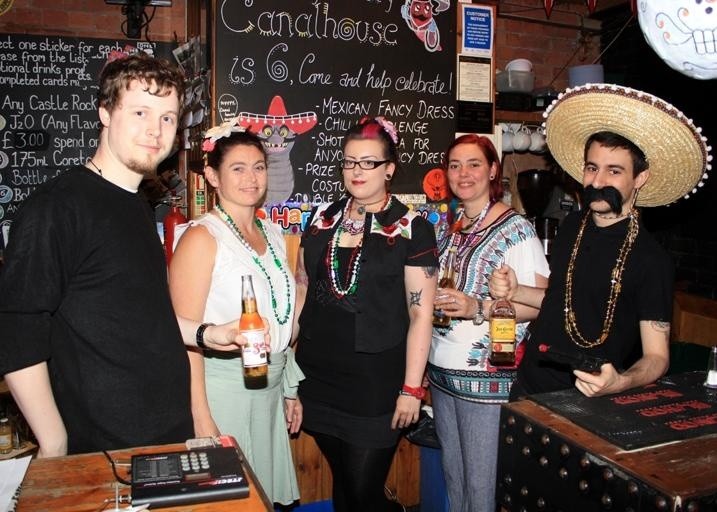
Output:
[[196, 320, 215, 354], [472, 297, 486, 326]]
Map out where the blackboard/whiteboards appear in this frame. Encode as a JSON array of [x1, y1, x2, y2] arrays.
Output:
[[0, 31, 181, 231], [212, 2, 457, 205]]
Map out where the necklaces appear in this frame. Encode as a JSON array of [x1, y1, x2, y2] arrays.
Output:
[[325, 190, 394, 302], [215, 202, 291, 326], [87, 158, 104, 179], [440, 200, 492, 272], [563, 208, 641, 350]]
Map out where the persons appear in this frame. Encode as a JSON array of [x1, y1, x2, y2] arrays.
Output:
[[288, 121, 441, 512], [508, 130, 674, 404], [0, 54, 196, 459], [169, 124, 306, 512], [424, 133, 552, 512]]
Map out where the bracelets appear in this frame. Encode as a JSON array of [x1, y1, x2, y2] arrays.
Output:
[[398, 383, 429, 402]]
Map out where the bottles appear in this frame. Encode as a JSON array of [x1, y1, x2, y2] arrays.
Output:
[[238, 274, 269, 391], [432, 248, 458, 329], [487, 257, 517, 367], [0, 418, 12, 455]]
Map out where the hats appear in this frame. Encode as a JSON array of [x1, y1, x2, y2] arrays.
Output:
[[540, 81, 712, 208], [239, 96, 317, 135]]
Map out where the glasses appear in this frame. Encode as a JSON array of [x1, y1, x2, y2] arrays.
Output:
[[339, 158, 390, 171]]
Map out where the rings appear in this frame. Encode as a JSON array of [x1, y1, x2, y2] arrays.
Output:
[[452, 296, 458, 304]]
